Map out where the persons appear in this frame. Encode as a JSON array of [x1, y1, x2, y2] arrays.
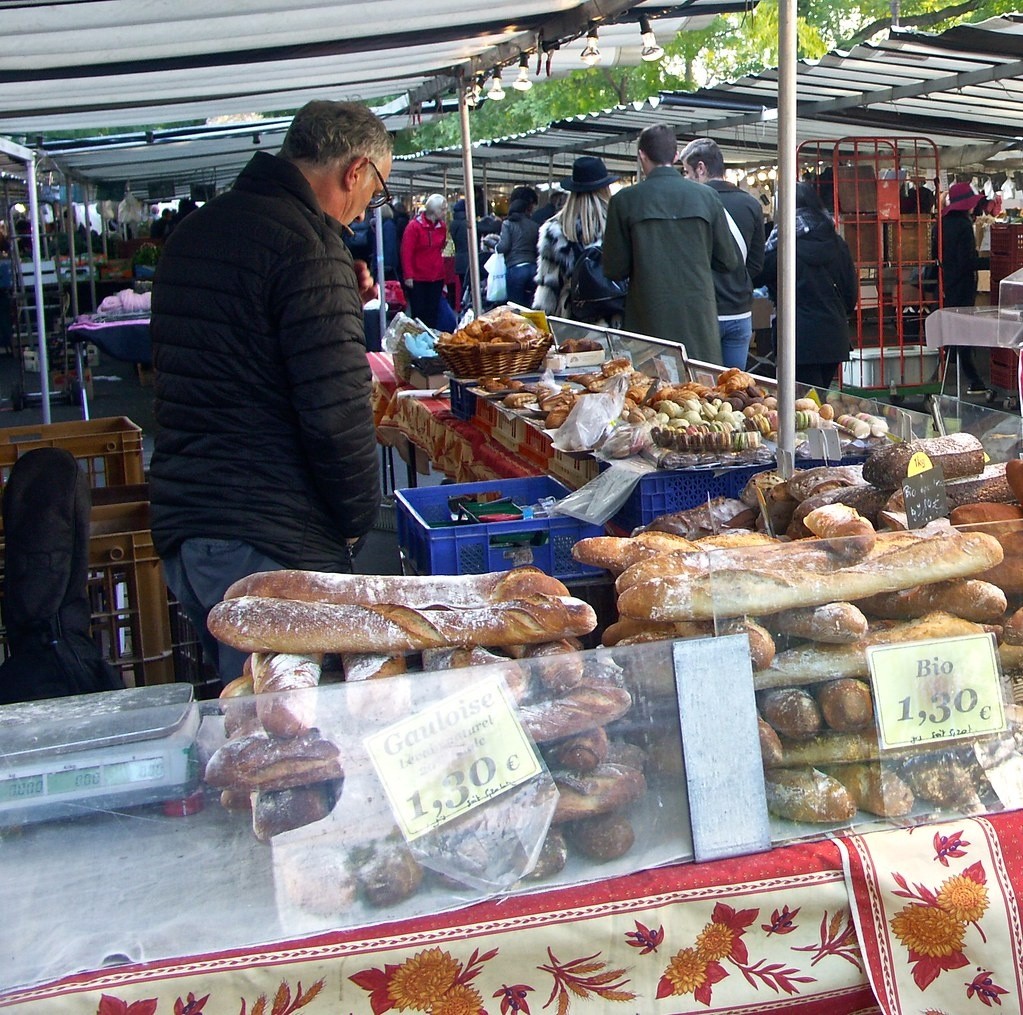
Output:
[[753, 182, 859, 385], [601, 124, 741, 367], [448, 199, 502, 297], [931, 183, 991, 394], [531, 157, 630, 329], [145, 99, 393, 687], [400, 194, 455, 328], [16, 220, 32, 250], [531, 192, 568, 226], [346, 202, 409, 281], [680, 138, 765, 372], [109, 199, 198, 246], [473, 184, 493, 217], [496, 187, 541, 308]]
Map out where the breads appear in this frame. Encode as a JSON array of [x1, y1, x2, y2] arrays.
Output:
[[477, 359, 890, 467], [437, 310, 603, 353], [203, 432, 1023, 914]]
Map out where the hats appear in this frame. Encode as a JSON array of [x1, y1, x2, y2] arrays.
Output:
[[560, 157, 620, 191], [453, 200, 465, 212], [943, 183, 984, 216]]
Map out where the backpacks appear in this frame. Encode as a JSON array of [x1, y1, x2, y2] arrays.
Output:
[[565, 238, 630, 322]]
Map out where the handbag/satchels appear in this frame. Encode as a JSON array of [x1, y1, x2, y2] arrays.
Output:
[[483, 245, 507, 302], [752, 298, 773, 330]]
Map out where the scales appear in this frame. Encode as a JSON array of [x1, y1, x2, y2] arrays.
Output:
[[1, 683, 201, 827]]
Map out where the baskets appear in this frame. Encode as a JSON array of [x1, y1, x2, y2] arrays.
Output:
[[449, 376, 864, 533], [431, 334, 552, 379], [392, 353, 421, 382], [391, 474, 614, 582], [397, 323, 435, 354]]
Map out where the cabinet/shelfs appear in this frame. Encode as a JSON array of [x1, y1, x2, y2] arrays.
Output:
[[796, 135, 943, 397]]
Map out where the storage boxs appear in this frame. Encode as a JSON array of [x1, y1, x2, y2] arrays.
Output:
[[391, 366, 874, 581], [988, 223, 1023, 391], [0, 417, 225, 696], [842, 344, 944, 386]]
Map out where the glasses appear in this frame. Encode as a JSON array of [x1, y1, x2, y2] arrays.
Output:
[[368, 160, 394, 210]]
